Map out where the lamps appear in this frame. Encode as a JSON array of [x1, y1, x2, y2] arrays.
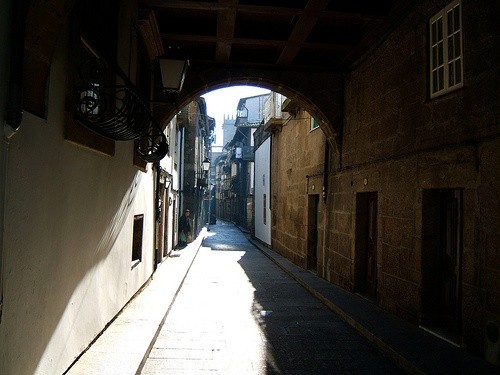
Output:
[[155, 42, 192, 93], [164, 175, 171, 189], [201, 158, 211, 178]]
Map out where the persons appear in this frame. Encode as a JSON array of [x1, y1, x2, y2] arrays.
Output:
[[179, 209, 190, 246]]
